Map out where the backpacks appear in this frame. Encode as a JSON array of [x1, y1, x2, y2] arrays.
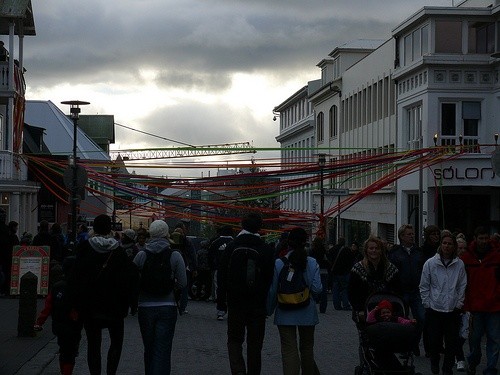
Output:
[[277, 256, 311, 310], [141, 247, 181, 297], [227, 241, 264, 309]]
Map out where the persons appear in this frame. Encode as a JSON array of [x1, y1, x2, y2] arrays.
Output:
[[266, 228, 323, 375], [128, 219, 187, 375], [220, 214, 274, 375], [67, 214, 129, 375], [36, 282, 83, 375], [0, 221, 152, 318], [309, 224, 500, 375], [168, 223, 292, 320]]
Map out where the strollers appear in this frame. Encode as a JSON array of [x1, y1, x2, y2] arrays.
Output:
[[353, 292, 423, 375]]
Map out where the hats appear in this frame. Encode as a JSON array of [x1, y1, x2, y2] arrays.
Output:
[[149, 220, 169, 237], [241, 214, 262, 233], [122, 229, 136, 240]]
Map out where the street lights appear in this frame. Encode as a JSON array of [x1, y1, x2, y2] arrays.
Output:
[[312, 152, 331, 237], [62, 101, 90, 246]]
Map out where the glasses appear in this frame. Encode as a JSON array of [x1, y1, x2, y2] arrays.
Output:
[[429, 233, 440, 235]]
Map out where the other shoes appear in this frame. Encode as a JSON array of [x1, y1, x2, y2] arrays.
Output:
[[217, 315, 223, 321], [399, 353, 406, 359], [443, 370, 453, 375], [455, 361, 465, 371], [432, 365, 440, 375], [414, 348, 421, 356]]
